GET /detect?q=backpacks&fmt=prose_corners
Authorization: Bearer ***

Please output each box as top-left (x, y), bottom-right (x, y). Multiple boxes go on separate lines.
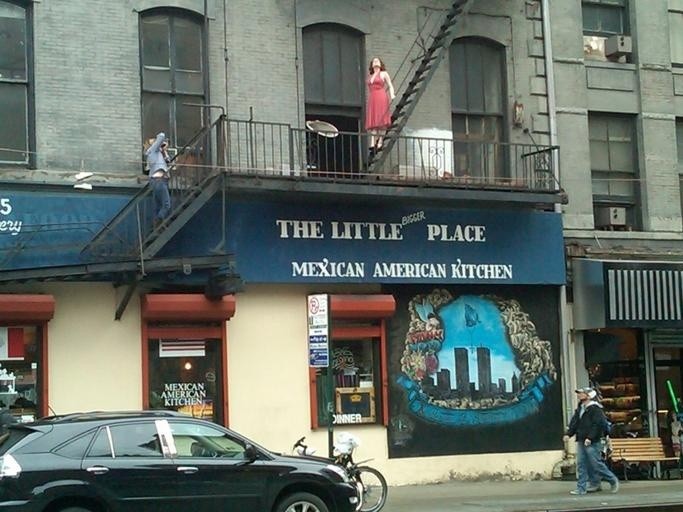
top-left (601, 416), bottom-right (613, 436)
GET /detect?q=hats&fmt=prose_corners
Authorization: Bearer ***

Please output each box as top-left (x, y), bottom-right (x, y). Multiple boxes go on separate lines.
top-left (575, 386), bottom-right (599, 398)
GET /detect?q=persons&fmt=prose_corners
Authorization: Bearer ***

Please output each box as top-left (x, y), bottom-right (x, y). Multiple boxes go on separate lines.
top-left (365, 55), bottom-right (396, 151)
top-left (585, 433), bottom-right (613, 493)
top-left (142, 130), bottom-right (175, 233)
top-left (567, 385), bottom-right (620, 494)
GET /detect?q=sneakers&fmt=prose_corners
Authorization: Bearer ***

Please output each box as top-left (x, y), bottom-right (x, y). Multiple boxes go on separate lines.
top-left (368, 146), bottom-right (383, 151)
top-left (151, 218), bottom-right (165, 234)
top-left (567, 483), bottom-right (601, 495)
top-left (610, 481), bottom-right (619, 494)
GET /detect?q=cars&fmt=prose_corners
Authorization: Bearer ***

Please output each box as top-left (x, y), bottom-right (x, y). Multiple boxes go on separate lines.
top-left (0, 410), bottom-right (357, 512)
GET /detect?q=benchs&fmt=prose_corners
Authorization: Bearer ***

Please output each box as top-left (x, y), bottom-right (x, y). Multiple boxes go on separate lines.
top-left (601, 436), bottom-right (681, 480)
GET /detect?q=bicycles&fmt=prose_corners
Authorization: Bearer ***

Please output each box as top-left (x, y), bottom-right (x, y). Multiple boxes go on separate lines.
top-left (284, 432), bottom-right (387, 511)
